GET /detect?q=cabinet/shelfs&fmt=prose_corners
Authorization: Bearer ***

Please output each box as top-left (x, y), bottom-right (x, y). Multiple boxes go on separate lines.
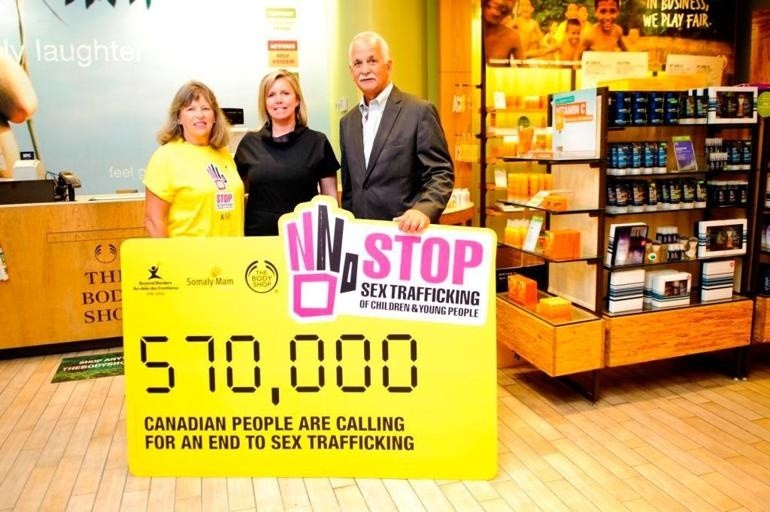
top-left (475, 77), bottom-right (770, 403)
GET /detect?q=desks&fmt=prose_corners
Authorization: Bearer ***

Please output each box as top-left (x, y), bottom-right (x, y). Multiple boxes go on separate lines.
top-left (438, 202), bottom-right (475, 226)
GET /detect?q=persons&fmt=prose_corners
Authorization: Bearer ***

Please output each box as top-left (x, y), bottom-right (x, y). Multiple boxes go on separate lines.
top-left (234, 68), bottom-right (341, 236)
top-left (338, 32), bottom-right (456, 233)
top-left (142, 79), bottom-right (247, 238)
top-left (481, 0), bottom-right (630, 64)
top-left (0, 39), bottom-right (37, 282)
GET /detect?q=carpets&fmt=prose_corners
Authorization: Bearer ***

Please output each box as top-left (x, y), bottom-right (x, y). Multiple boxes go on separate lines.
top-left (51, 352), bottom-right (124, 384)
